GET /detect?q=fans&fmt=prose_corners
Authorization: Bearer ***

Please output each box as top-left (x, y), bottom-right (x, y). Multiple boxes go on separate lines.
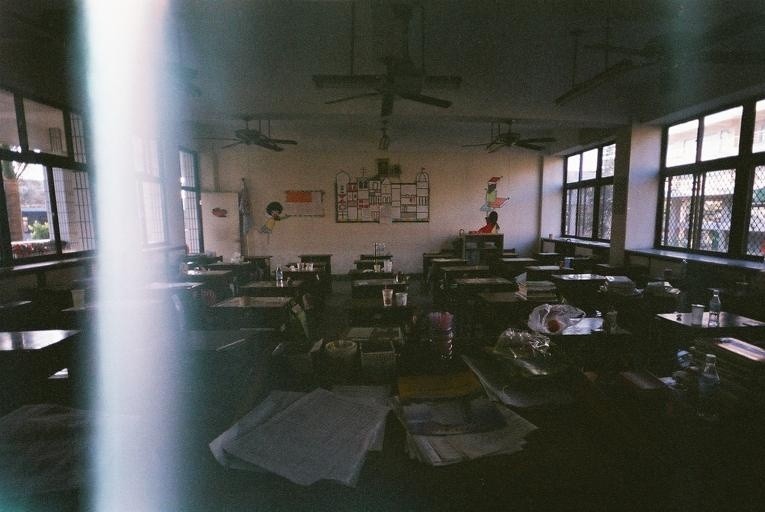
top-left (192, 116), bottom-right (299, 156)
top-left (312, 57), bottom-right (464, 119)
top-left (461, 118), bottom-right (562, 158)
top-left (595, 1), bottom-right (765, 83)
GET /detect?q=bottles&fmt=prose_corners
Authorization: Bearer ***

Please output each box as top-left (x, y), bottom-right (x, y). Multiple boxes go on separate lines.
top-left (668, 345), bottom-right (720, 423)
top-left (690, 283), bottom-right (725, 326)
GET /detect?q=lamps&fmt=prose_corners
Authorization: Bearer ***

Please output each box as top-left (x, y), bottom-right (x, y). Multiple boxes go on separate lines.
top-left (312, 0), bottom-right (465, 92)
top-left (552, 14), bottom-right (637, 108)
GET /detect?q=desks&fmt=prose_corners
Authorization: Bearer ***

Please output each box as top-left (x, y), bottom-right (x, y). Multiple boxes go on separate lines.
top-left (154, 254), bottom-right (331, 385)
top-left (423, 252), bottom-right (764, 429)
top-left (349, 255), bottom-right (412, 366)
top-left (1, 276), bottom-right (95, 404)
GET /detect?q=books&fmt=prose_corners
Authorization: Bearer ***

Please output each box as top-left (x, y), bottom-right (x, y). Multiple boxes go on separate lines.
top-left (706, 336), bottom-right (765, 412)
top-left (599, 275), bottom-right (642, 300)
top-left (397, 370), bottom-right (480, 403)
top-left (516, 279), bottom-right (558, 304)
top-left (618, 368), bottom-right (667, 400)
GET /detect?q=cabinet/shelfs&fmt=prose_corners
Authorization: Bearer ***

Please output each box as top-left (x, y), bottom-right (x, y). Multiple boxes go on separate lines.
top-left (461, 232), bottom-right (505, 264)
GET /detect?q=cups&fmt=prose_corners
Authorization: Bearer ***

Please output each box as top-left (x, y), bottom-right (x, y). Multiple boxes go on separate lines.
top-left (187, 253), bottom-right (314, 286)
top-left (373, 257), bottom-right (410, 309)
top-left (68, 289), bottom-right (86, 310)
